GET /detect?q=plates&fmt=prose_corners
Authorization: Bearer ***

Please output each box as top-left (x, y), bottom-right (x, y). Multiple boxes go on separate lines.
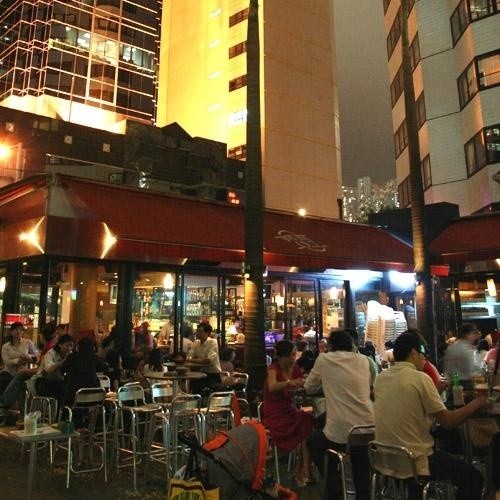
top-left (176, 367), bottom-right (188, 372)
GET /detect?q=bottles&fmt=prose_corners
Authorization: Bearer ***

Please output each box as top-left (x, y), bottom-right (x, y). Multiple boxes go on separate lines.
top-left (453, 371), bottom-right (464, 408)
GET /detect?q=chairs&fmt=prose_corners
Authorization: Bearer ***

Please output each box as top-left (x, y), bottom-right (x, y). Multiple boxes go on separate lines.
top-left (52, 388), bottom-right (107, 489)
top-left (152, 382), bottom-right (183, 412)
top-left (200, 391), bottom-right (234, 446)
top-left (108, 384), bottom-right (164, 489)
top-left (368, 440), bottom-right (458, 500)
top-left (322, 425), bottom-right (375, 499)
top-left (213, 371), bottom-right (252, 433)
top-left (119, 382), bottom-right (141, 449)
top-left (220, 371), bottom-right (230, 383)
top-left (98, 375), bottom-right (118, 442)
top-left (258, 400), bottom-right (296, 484)
top-left (141, 394), bottom-right (202, 491)
top-left (22, 389), bottom-right (57, 464)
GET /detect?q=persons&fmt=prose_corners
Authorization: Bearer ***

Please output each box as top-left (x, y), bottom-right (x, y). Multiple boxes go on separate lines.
top-left (264, 322), bottom-right (499, 499)
top-left (0, 313), bottom-right (244, 452)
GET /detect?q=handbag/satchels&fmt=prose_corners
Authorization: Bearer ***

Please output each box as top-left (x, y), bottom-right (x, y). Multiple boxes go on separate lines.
top-left (167, 436), bottom-right (220, 500)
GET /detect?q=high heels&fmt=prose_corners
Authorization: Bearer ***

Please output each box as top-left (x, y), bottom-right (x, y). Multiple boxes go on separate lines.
top-left (292, 466), bottom-right (316, 489)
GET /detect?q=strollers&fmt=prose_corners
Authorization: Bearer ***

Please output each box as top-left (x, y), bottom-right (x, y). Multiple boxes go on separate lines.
top-left (167, 420), bottom-right (301, 495)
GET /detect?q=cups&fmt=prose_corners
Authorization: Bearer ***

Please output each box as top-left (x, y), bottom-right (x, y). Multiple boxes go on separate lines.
top-left (24, 415), bottom-right (36, 435)
top-left (473, 384), bottom-right (489, 412)
top-left (163, 362), bottom-right (176, 375)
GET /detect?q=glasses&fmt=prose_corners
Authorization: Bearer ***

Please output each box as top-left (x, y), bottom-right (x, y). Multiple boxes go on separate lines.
top-left (418, 350), bottom-right (429, 359)
